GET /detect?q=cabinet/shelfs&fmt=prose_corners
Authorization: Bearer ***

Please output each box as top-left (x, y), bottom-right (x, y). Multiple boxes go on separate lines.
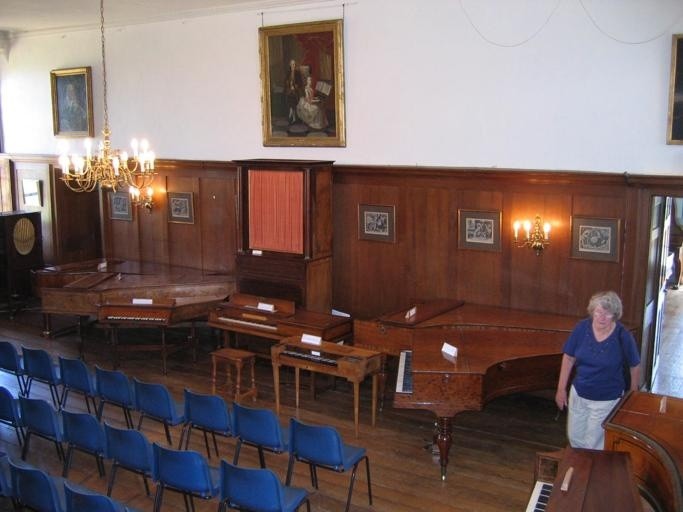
top-left (234, 252), bottom-right (307, 300)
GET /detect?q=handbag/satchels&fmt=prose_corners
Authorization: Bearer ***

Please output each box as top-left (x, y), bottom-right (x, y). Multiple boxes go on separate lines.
top-left (624, 360), bottom-right (631, 391)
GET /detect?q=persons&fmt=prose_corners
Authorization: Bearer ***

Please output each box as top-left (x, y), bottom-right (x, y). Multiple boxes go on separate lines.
top-left (285, 59), bottom-right (306, 126)
top-left (296, 77), bottom-right (328, 129)
top-left (555, 291), bottom-right (640, 451)
top-left (59, 84), bottom-right (87, 131)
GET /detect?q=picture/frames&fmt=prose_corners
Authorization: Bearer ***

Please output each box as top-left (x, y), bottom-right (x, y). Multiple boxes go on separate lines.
top-left (107, 192), bottom-right (133, 221)
top-left (166, 190), bottom-right (195, 225)
top-left (50, 66), bottom-right (95, 138)
top-left (457, 209), bottom-right (502, 254)
top-left (667, 35), bottom-right (683, 146)
top-left (358, 204), bottom-right (396, 245)
top-left (569, 216), bottom-right (622, 263)
top-left (20, 178), bottom-right (43, 208)
top-left (259, 19), bottom-right (347, 148)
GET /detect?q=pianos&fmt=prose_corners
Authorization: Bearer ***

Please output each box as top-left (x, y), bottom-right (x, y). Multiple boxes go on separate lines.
top-left (270, 334), bottom-right (384, 439)
top-left (98, 296), bottom-right (228, 375)
top-left (355, 297), bottom-right (639, 482)
top-left (523, 447), bottom-right (638, 511)
top-left (32, 256), bottom-right (235, 344)
top-left (208, 293), bottom-right (352, 400)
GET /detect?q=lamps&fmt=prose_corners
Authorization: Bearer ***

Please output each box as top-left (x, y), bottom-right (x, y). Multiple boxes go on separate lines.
top-left (57, 0), bottom-right (157, 193)
top-left (512, 210), bottom-right (552, 256)
top-left (130, 186), bottom-right (156, 212)
